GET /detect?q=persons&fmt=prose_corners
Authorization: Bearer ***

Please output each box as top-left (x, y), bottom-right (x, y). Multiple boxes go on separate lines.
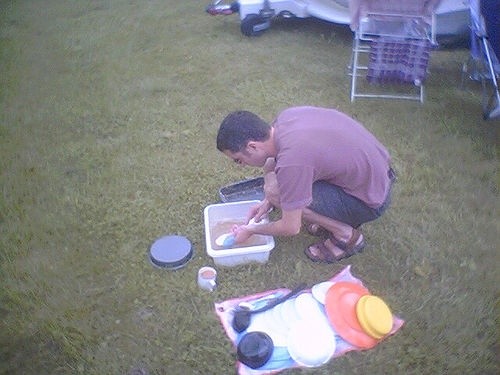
top-left (212, 105), bottom-right (396, 263)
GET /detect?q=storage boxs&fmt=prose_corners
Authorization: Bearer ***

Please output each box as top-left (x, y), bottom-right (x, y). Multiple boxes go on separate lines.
top-left (204, 199), bottom-right (275, 268)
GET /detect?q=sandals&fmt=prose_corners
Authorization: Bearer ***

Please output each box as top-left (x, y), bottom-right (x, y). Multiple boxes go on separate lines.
top-left (307, 224), bottom-right (361, 238)
top-left (304, 228), bottom-right (367, 263)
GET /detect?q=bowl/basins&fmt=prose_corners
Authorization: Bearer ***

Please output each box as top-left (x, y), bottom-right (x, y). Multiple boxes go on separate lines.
top-left (236, 331), bottom-right (275, 370)
top-left (285, 319), bottom-right (336, 366)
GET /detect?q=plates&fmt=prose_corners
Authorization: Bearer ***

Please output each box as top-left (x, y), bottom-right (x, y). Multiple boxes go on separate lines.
top-left (149, 236), bottom-right (193, 269)
top-left (232, 281), bottom-right (394, 370)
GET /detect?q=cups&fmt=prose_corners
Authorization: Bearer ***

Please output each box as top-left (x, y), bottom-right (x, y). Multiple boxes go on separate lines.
top-left (198, 267), bottom-right (217, 292)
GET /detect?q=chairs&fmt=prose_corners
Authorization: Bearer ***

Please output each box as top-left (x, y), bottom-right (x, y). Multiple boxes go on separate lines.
top-left (346, 0), bottom-right (441, 104)
